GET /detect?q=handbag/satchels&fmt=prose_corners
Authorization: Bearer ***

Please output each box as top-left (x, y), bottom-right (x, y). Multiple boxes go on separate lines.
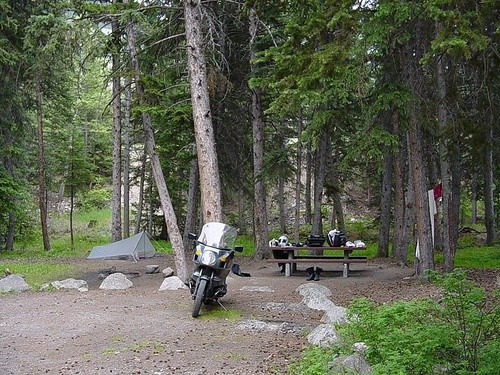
top-left (327, 228), bottom-right (346, 247)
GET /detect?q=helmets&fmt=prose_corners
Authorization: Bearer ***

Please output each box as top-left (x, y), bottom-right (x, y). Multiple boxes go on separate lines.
top-left (278, 236), bottom-right (288, 246)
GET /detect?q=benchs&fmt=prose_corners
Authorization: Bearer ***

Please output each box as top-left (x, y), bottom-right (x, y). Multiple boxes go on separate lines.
top-left (293, 256), bottom-right (367, 273)
top-left (266, 259), bottom-right (367, 278)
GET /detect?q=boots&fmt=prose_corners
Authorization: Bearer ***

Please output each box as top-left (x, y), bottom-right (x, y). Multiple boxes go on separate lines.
top-left (314, 267), bottom-right (322, 281)
top-left (306, 267), bottom-right (315, 281)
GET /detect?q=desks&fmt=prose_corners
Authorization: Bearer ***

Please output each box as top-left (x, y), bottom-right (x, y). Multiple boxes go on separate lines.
top-left (266, 246), bottom-right (368, 274)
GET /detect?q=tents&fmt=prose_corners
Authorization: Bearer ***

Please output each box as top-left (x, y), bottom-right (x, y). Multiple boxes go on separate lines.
top-left (84, 230), bottom-right (156, 261)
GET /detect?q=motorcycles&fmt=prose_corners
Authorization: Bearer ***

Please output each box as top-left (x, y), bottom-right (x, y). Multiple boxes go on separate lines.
top-left (184, 222), bottom-right (243, 318)
